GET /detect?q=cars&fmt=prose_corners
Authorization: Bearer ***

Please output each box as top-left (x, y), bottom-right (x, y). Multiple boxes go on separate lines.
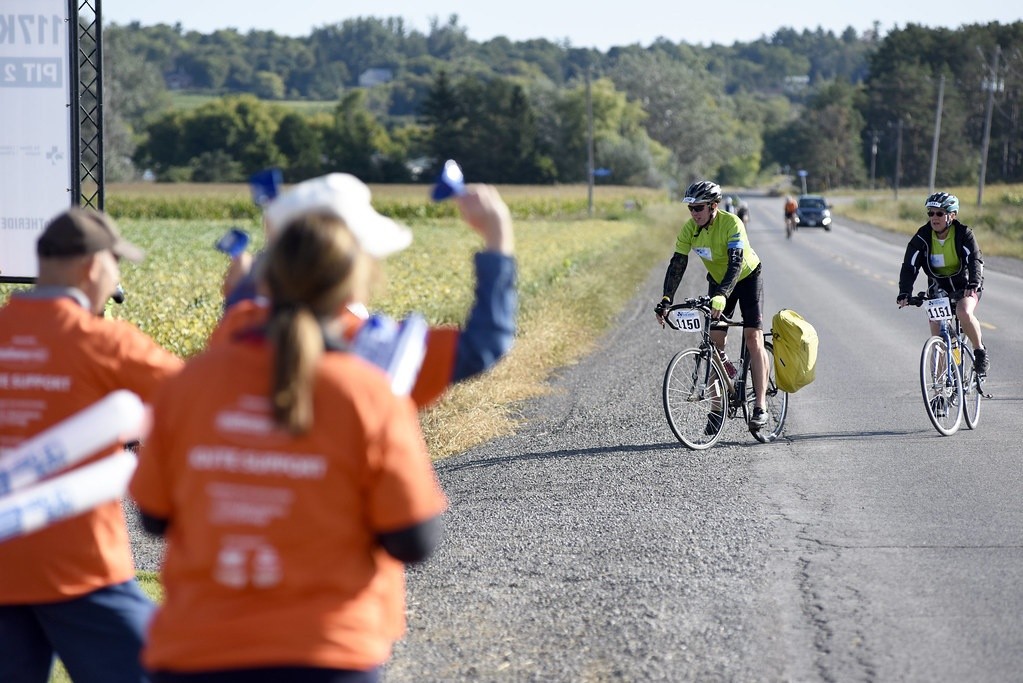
top-left (795, 195), bottom-right (834, 232)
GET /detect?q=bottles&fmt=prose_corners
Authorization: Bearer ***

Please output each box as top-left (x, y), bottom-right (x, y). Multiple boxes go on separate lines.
top-left (716, 347), bottom-right (737, 378)
top-left (951, 335), bottom-right (961, 365)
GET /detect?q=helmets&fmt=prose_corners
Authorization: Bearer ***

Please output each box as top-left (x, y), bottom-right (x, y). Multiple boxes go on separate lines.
top-left (681, 181), bottom-right (722, 203)
top-left (925, 192), bottom-right (959, 215)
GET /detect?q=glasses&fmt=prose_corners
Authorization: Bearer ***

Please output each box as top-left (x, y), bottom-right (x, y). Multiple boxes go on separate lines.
top-left (688, 203), bottom-right (713, 212)
top-left (927, 211), bottom-right (951, 217)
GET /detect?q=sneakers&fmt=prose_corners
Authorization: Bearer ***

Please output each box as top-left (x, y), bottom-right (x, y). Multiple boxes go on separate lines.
top-left (930, 396), bottom-right (945, 417)
top-left (748, 405), bottom-right (769, 427)
top-left (704, 411), bottom-right (724, 435)
top-left (974, 344), bottom-right (990, 374)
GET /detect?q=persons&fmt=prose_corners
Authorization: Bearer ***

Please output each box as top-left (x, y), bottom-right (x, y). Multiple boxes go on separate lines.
top-left (896, 192), bottom-right (990, 417)
top-left (784, 197), bottom-right (798, 234)
top-left (724, 196), bottom-right (750, 224)
top-left (656, 180), bottom-right (769, 435)
top-left (0, 205), bottom-right (185, 683)
top-left (129, 172), bottom-right (517, 683)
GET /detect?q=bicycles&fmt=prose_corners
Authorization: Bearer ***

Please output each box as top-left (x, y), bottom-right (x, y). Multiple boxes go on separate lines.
top-left (653, 294), bottom-right (790, 451)
top-left (783, 211), bottom-right (798, 238)
top-left (896, 286), bottom-right (993, 437)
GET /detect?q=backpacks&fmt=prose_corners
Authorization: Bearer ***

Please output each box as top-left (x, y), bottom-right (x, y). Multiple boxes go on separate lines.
top-left (771, 310), bottom-right (818, 393)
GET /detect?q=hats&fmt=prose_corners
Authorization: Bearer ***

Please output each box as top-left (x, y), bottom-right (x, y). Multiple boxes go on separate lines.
top-left (264, 173), bottom-right (414, 257)
top-left (38, 208), bottom-right (144, 262)
